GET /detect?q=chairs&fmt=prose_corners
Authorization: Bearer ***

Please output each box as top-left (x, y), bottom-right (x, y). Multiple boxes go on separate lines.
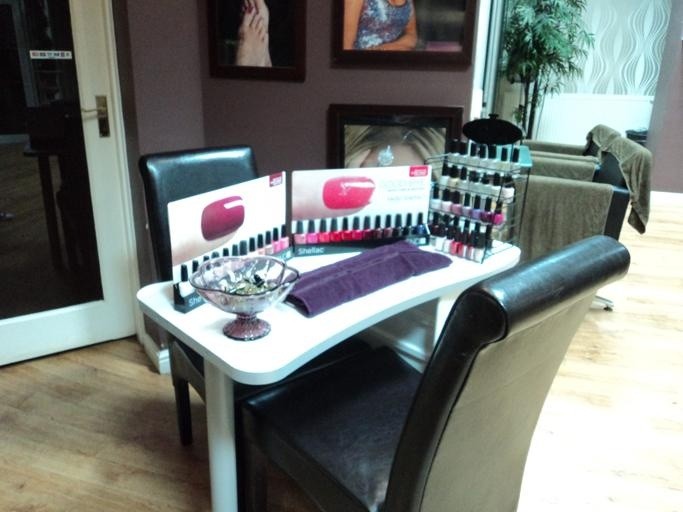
top-left (139, 147), bottom-right (373, 487)
top-left (510, 125), bottom-right (651, 266)
top-left (261, 234), bottom-right (631, 512)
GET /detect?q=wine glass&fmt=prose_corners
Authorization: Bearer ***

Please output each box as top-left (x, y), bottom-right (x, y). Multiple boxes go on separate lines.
top-left (189, 255), bottom-right (299, 341)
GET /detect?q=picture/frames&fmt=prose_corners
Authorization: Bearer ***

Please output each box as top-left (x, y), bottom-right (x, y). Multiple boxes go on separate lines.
top-left (207, 0), bottom-right (477, 83)
top-left (327, 104), bottom-right (463, 167)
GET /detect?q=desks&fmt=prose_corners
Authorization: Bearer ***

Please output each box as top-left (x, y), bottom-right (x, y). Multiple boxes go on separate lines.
top-left (137, 223), bottom-right (522, 512)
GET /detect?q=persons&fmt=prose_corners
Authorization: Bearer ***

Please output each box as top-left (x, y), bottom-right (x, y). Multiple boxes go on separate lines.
top-left (232, 0), bottom-right (272, 67)
top-left (345, 123), bottom-right (446, 168)
top-left (342, 0), bottom-right (417, 50)
